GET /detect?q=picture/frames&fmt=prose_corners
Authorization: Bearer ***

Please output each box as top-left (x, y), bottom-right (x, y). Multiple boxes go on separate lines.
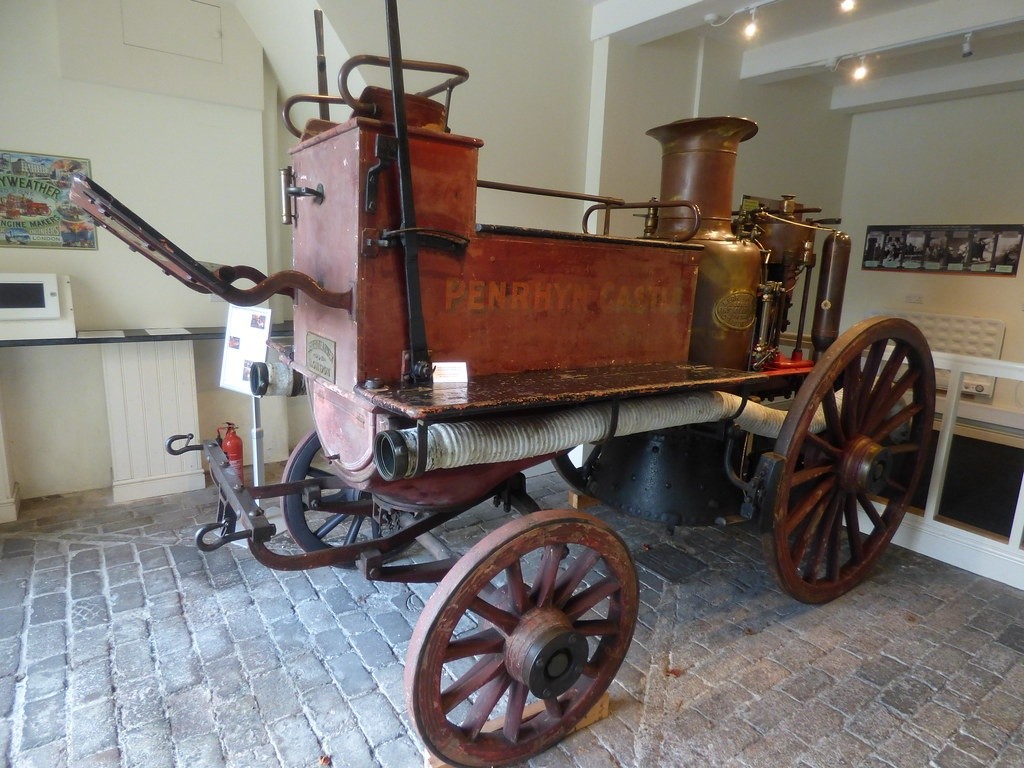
top-left (860, 224), bottom-right (1024, 274)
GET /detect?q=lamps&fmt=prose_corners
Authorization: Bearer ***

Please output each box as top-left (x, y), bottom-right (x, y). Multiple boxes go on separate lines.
top-left (853, 55), bottom-right (868, 81)
top-left (744, 8), bottom-right (759, 38)
top-left (961, 32), bottom-right (973, 58)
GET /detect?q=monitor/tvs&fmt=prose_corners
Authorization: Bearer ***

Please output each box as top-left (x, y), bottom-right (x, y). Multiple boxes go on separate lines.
top-left (0, 274), bottom-right (60, 321)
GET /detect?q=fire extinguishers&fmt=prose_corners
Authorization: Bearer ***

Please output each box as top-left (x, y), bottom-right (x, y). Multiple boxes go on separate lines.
top-left (216, 421), bottom-right (244, 487)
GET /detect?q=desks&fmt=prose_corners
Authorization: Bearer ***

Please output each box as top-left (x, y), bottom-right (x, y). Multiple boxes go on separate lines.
top-left (0, 320), bottom-right (294, 523)
